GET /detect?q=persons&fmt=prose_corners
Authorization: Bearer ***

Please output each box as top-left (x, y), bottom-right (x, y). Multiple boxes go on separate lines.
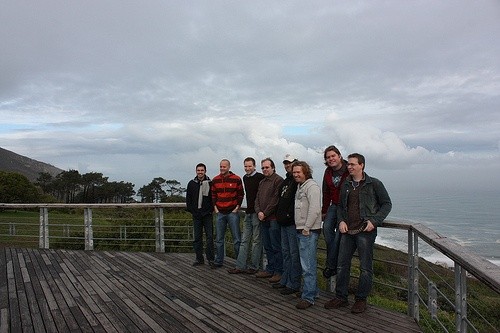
top-left (183, 144), bottom-right (392, 313)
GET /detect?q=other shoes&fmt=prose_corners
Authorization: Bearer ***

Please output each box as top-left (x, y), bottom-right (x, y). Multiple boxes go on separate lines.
top-left (324, 297), bottom-right (348, 309)
top-left (352, 300), bottom-right (367, 312)
top-left (228, 268), bottom-right (247, 274)
top-left (256, 272), bottom-right (272, 277)
top-left (245, 269), bottom-right (258, 274)
top-left (280, 288), bottom-right (298, 295)
top-left (296, 292), bottom-right (320, 298)
top-left (208, 260), bottom-right (224, 268)
top-left (272, 283), bottom-right (286, 288)
top-left (193, 260), bottom-right (204, 265)
top-left (295, 300), bottom-right (312, 308)
top-left (271, 274), bottom-right (282, 282)
top-left (323, 267), bottom-right (337, 279)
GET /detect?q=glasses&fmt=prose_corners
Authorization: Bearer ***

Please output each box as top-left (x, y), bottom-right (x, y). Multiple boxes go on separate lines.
top-left (261, 166), bottom-right (270, 169)
top-left (346, 163), bottom-right (358, 166)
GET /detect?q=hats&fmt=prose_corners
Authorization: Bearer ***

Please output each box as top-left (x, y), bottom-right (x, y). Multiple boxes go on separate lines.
top-left (283, 153), bottom-right (297, 163)
